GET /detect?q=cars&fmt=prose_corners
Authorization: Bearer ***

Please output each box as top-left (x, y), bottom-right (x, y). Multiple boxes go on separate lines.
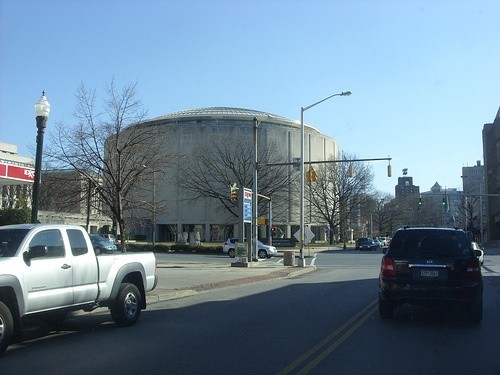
top-left (356, 236), bottom-right (392, 254)
top-left (87, 233), bottom-right (118, 255)
top-left (470, 243), bottom-right (484, 264)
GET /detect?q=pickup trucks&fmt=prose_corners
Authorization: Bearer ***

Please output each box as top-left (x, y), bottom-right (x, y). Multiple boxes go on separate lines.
top-left (0, 224), bottom-right (158, 375)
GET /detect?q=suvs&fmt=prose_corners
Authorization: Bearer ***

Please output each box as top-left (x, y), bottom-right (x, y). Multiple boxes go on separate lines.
top-left (223, 237), bottom-right (277, 258)
top-left (378, 225), bottom-right (484, 322)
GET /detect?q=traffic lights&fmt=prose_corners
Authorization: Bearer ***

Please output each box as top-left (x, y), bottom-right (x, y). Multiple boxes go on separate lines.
top-left (270, 227), bottom-right (278, 232)
top-left (418, 198), bottom-right (422, 205)
top-left (443, 198), bottom-right (447, 206)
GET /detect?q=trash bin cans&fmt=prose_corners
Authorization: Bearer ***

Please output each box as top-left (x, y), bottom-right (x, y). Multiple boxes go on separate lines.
top-left (284, 251), bottom-right (295, 266)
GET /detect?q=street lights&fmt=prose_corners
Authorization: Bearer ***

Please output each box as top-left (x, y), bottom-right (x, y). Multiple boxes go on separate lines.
top-left (297, 91), bottom-right (352, 268)
top-left (31, 90), bottom-right (51, 224)
